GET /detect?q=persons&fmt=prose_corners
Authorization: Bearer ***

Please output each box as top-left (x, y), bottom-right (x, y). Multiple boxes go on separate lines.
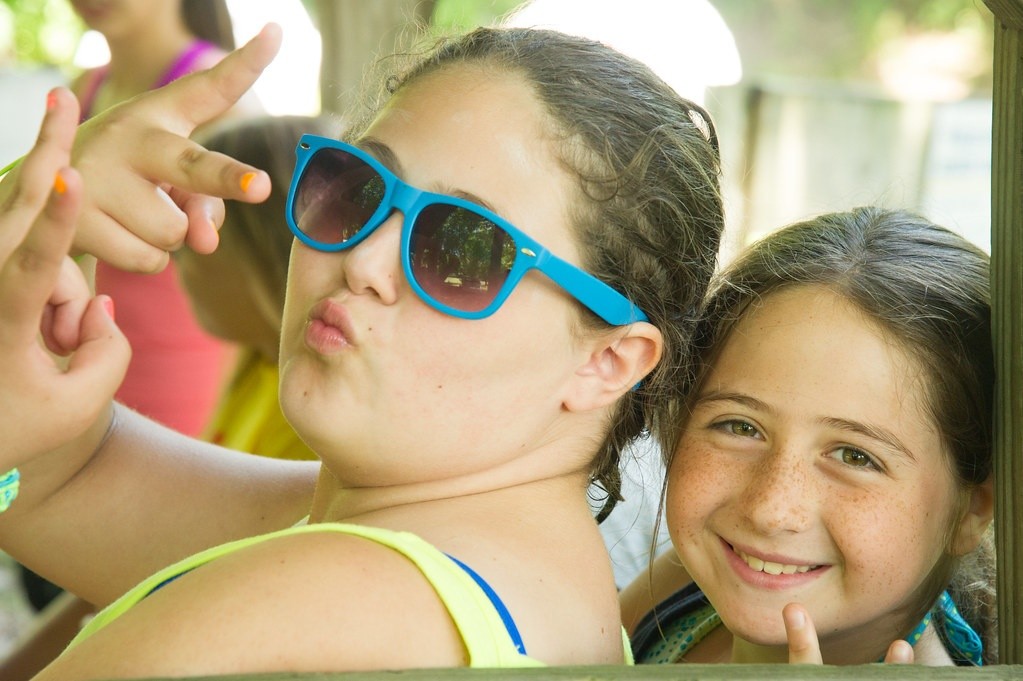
top-left (617, 205), bottom-right (997, 666)
top-left (58, 0), bottom-right (261, 437)
top-left (1, 114), bottom-right (359, 678)
top-left (2, 23), bottom-right (726, 680)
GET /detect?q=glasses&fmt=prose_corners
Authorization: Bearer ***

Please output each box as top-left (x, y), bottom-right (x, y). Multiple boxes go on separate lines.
top-left (289, 131), bottom-right (649, 397)
